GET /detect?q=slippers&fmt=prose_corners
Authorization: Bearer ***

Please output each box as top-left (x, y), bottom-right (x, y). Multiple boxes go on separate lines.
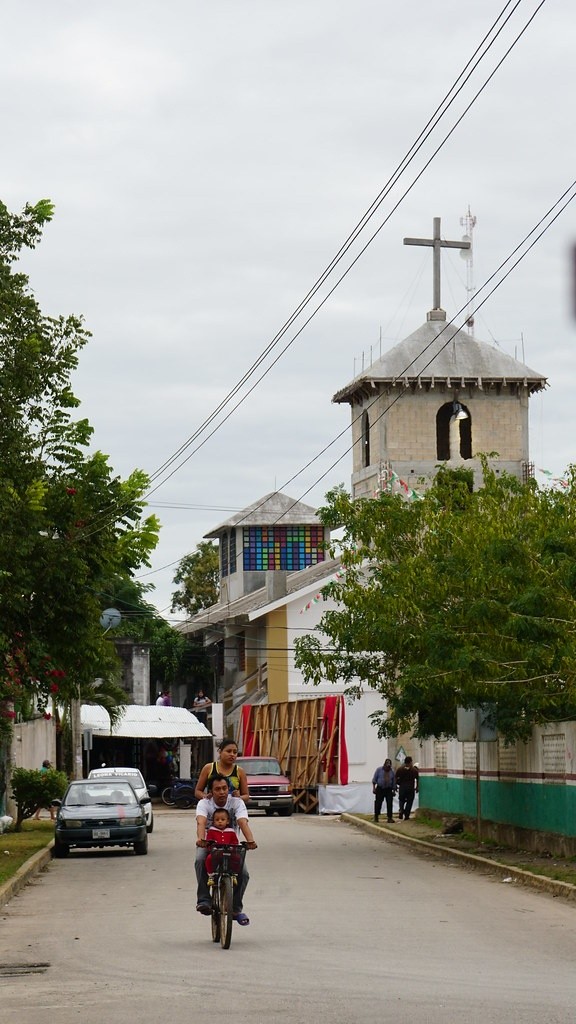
top-left (196, 901), bottom-right (213, 916)
top-left (235, 913), bottom-right (250, 926)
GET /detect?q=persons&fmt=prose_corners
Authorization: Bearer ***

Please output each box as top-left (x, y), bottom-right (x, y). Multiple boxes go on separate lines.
top-left (204, 808), bottom-right (240, 886)
top-left (156, 687), bottom-right (172, 706)
top-left (193, 690), bottom-right (211, 728)
top-left (194, 775), bottom-right (256, 926)
top-left (195, 740), bottom-right (249, 803)
top-left (372, 759), bottom-right (397, 823)
top-left (33, 760), bottom-right (57, 820)
top-left (394, 756), bottom-right (419, 820)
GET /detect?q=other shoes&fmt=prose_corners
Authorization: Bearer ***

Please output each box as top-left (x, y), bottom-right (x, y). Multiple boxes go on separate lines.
top-left (399, 812), bottom-right (403, 819)
top-left (374, 815), bottom-right (378, 822)
top-left (404, 817), bottom-right (410, 820)
top-left (388, 817), bottom-right (395, 823)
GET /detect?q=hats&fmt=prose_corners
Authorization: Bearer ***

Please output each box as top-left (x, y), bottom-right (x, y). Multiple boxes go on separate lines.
top-left (383, 759), bottom-right (391, 772)
top-left (43, 760), bottom-right (52, 765)
top-left (405, 757), bottom-right (412, 764)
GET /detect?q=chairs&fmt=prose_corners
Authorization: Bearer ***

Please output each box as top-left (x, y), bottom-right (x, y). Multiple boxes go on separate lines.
top-left (111, 791), bottom-right (124, 802)
top-left (259, 766), bottom-right (270, 772)
top-left (78, 792), bottom-right (91, 803)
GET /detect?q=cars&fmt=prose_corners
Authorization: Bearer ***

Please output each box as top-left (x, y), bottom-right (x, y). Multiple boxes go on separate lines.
top-left (87, 767), bottom-right (157, 833)
top-left (233, 756), bottom-right (295, 816)
top-left (51, 779), bottom-right (148, 857)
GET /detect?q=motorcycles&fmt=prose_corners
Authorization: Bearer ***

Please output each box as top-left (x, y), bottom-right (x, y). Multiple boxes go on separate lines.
top-left (171, 778), bottom-right (199, 810)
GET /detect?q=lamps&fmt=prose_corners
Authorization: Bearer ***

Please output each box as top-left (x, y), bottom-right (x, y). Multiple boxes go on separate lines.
top-left (453, 403), bottom-right (468, 420)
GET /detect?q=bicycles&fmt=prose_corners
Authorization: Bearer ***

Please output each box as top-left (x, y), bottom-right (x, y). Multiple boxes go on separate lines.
top-left (160, 785), bottom-right (176, 806)
top-left (198, 839), bottom-right (255, 950)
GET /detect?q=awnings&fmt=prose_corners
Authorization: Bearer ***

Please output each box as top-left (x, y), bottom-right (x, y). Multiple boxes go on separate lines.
top-left (47, 706), bottom-right (213, 738)
